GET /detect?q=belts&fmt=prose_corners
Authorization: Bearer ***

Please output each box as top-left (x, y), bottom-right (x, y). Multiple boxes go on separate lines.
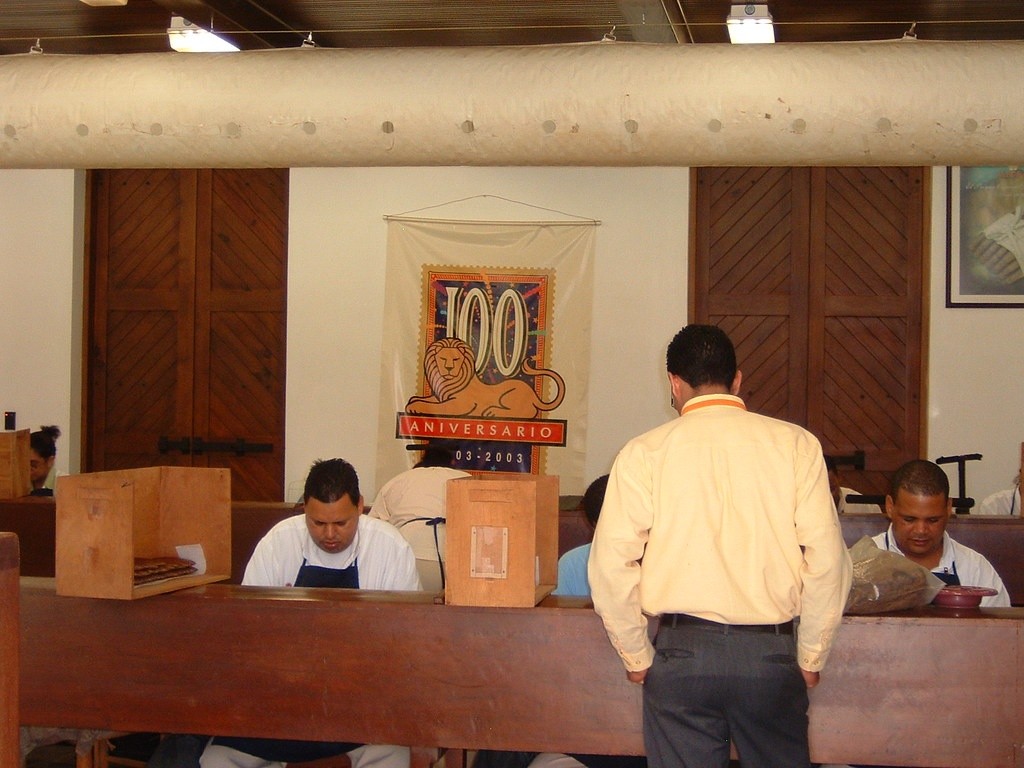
top-left (659, 613), bottom-right (794, 635)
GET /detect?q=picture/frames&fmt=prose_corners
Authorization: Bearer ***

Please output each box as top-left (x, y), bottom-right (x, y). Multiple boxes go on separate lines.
top-left (944, 165), bottom-right (1024, 309)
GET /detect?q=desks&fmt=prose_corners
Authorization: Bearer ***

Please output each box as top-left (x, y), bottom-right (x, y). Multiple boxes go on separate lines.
top-left (1, 494), bottom-right (1024, 606)
top-left (21, 578), bottom-right (1024, 768)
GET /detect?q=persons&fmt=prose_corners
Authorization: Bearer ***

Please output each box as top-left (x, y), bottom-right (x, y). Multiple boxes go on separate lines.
top-left (199, 458), bottom-right (423, 768)
top-left (366, 443), bottom-right (473, 595)
top-left (979, 471), bottom-right (1021, 515)
top-left (587, 323), bottom-right (854, 768)
top-left (29, 425), bottom-right (71, 496)
top-left (823, 454), bottom-right (883, 515)
top-left (868, 459), bottom-right (1012, 609)
top-left (529, 473), bottom-right (648, 768)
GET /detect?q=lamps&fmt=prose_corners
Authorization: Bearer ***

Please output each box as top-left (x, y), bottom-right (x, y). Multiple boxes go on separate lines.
top-left (726, 5), bottom-right (776, 43)
top-left (165, 15), bottom-right (240, 52)
